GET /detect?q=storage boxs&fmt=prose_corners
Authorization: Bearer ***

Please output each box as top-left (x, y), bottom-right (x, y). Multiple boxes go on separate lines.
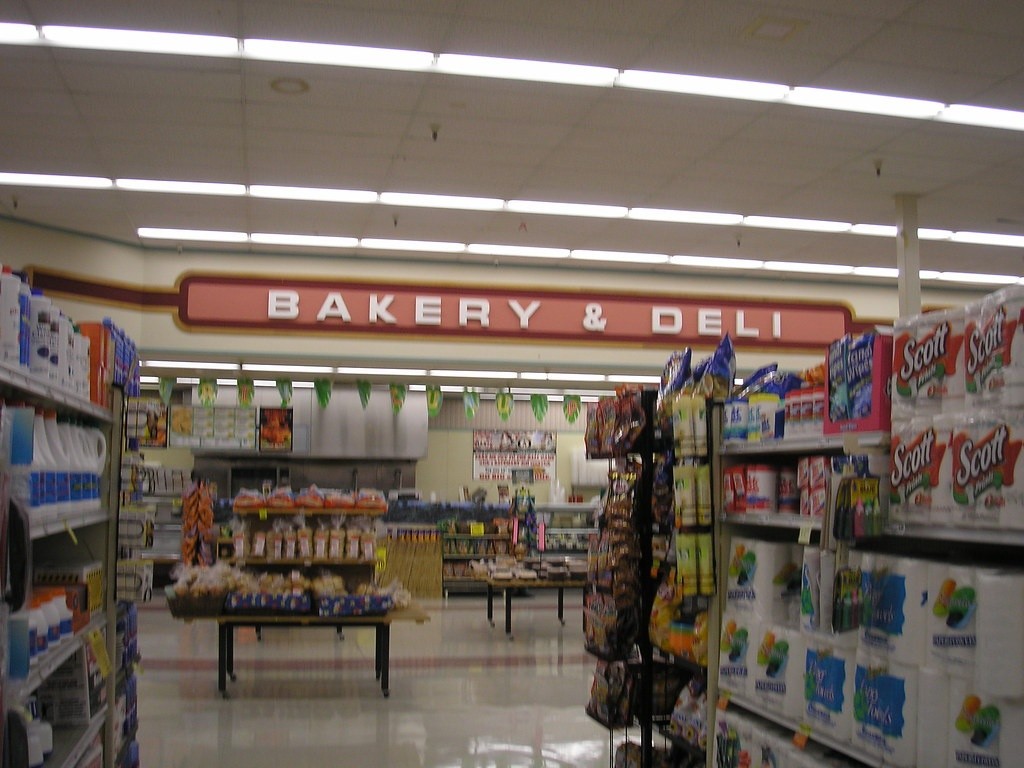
top-left (166, 585), bottom-right (394, 618)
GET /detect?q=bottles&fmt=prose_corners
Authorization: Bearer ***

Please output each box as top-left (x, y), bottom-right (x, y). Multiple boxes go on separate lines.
top-left (0, 264), bottom-right (110, 678)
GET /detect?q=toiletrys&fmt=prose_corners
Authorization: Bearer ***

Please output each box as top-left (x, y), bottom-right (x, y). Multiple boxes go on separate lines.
top-left (0, 264), bottom-right (140, 768)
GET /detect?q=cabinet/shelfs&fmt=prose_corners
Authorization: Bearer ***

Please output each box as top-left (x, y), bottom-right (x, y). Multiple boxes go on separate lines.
top-left (0, 365), bottom-right (127, 768)
top-left (585, 389), bottom-right (1024, 768)
top-left (442, 504), bottom-right (597, 599)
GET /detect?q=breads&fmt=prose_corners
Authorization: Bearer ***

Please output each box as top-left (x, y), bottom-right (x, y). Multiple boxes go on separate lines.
top-left (171, 528), bottom-right (378, 598)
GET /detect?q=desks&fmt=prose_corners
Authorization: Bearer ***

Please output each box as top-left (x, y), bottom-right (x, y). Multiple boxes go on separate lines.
top-left (483, 577), bottom-right (586, 633)
top-left (184, 602), bottom-right (432, 700)
top-left (232, 507), bottom-right (384, 585)
